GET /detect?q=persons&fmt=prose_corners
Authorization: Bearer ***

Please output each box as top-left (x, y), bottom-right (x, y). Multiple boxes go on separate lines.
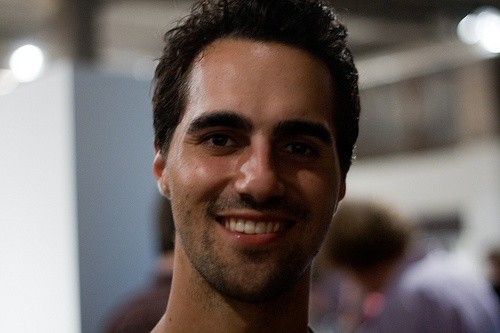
top-left (147, 1), bottom-right (361, 333)
top-left (308, 198), bottom-right (500, 332)
top-left (93, 191), bottom-right (175, 333)
